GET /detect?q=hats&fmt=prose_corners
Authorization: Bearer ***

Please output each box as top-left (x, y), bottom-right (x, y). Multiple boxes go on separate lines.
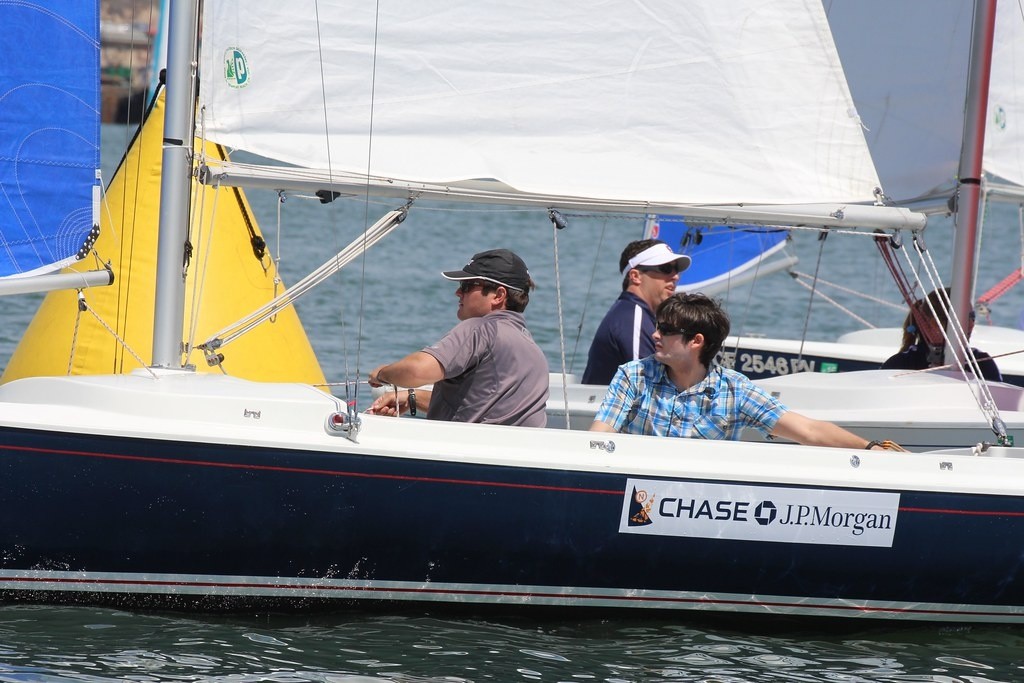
top-left (440, 248), bottom-right (530, 295)
top-left (623, 243), bottom-right (692, 281)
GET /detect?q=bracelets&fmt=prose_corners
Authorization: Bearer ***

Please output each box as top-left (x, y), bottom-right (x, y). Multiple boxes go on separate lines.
top-left (865, 439), bottom-right (881, 450)
top-left (408, 388), bottom-right (416, 416)
top-left (376, 368), bottom-right (391, 386)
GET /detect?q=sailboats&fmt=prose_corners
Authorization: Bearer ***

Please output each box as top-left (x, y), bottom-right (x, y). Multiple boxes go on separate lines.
top-left (1, 0), bottom-right (1024, 641)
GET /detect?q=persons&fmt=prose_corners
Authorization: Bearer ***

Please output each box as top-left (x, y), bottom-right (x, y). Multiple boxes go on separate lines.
top-left (581, 239), bottom-right (692, 385)
top-left (589, 293), bottom-right (910, 452)
top-left (368, 249), bottom-right (549, 427)
top-left (879, 287), bottom-right (1004, 381)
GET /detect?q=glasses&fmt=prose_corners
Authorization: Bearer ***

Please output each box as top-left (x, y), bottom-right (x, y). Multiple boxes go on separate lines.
top-left (459, 281), bottom-right (489, 293)
top-left (640, 264), bottom-right (679, 274)
top-left (656, 321), bottom-right (688, 335)
